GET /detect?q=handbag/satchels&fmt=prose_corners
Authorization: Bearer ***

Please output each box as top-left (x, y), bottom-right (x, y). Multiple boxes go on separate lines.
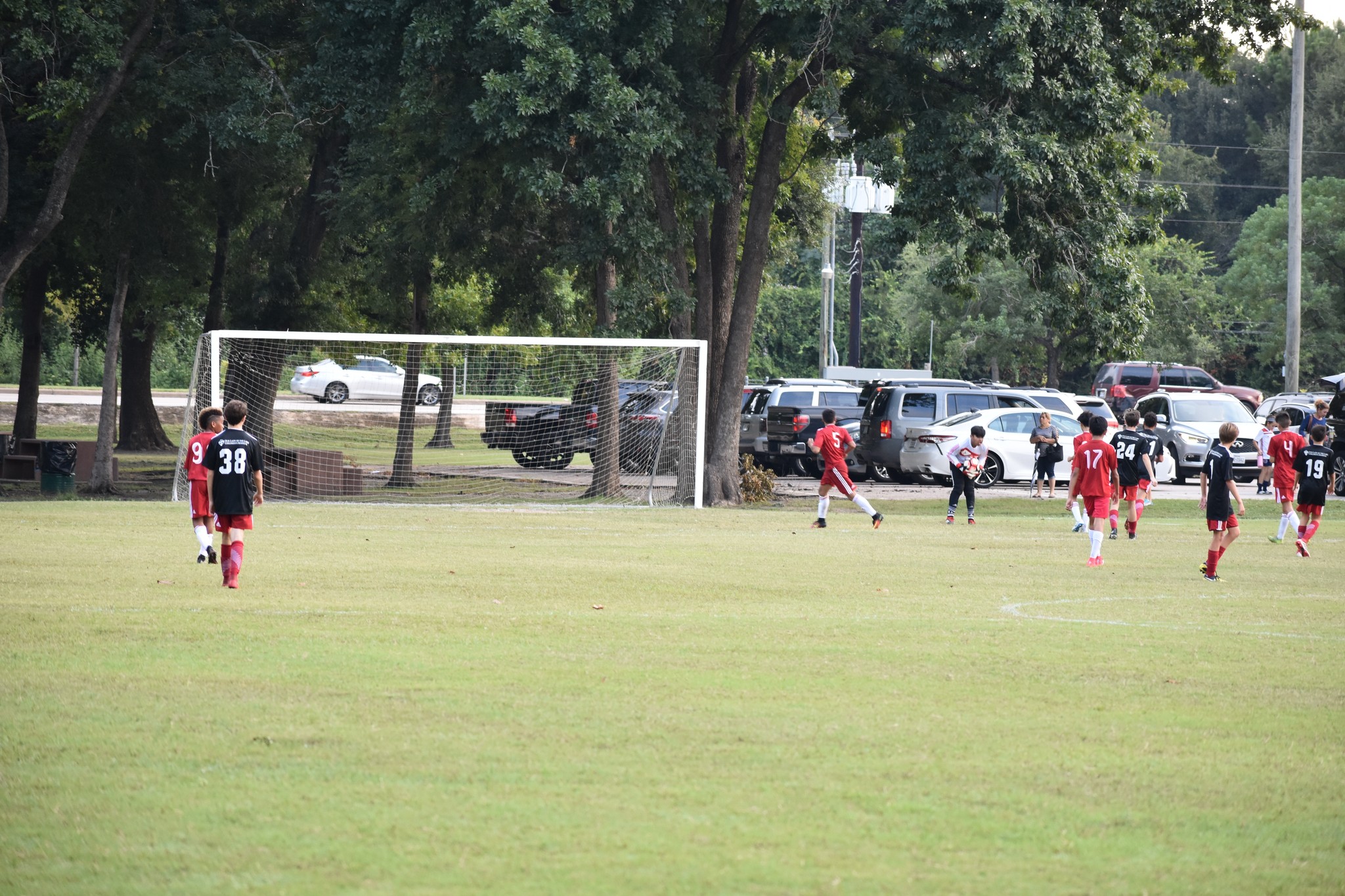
top-left (1304, 431), bottom-right (1310, 441)
top-left (1048, 446), bottom-right (1063, 462)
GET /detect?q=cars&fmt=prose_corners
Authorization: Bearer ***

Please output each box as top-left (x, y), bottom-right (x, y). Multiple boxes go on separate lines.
top-left (899, 407), bottom-right (1177, 489)
top-left (561, 374), bottom-right (1345, 496)
top-left (290, 355), bottom-right (443, 406)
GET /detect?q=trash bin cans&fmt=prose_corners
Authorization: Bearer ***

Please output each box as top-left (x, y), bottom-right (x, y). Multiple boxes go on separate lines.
top-left (40, 440), bottom-right (78, 493)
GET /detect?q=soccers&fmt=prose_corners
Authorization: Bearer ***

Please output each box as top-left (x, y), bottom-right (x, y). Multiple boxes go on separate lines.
top-left (963, 457), bottom-right (980, 474)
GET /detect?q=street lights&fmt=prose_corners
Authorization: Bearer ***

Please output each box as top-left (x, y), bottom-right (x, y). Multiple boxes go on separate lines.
top-left (841, 174), bottom-right (897, 367)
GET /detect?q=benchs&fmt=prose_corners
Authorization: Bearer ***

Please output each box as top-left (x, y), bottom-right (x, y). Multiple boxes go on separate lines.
top-left (342, 466), bottom-right (364, 496)
top-left (2, 455), bottom-right (37, 478)
top-left (263, 466), bottom-right (288, 495)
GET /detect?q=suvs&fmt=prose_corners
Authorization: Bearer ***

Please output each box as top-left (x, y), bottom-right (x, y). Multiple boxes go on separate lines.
top-left (1091, 361), bottom-right (1264, 425)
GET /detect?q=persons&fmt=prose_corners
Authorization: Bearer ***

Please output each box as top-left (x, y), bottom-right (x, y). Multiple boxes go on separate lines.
top-left (807, 409), bottom-right (883, 530)
top-left (183, 406), bottom-right (225, 564)
top-left (1252, 415), bottom-right (1277, 495)
top-left (1267, 412), bottom-right (1307, 543)
top-left (1198, 423), bottom-right (1245, 582)
top-left (945, 426), bottom-right (989, 526)
top-left (1030, 412), bottom-right (1059, 498)
top-left (1292, 424), bottom-right (1336, 558)
top-left (201, 399), bottom-right (264, 587)
top-left (1064, 408), bottom-right (1164, 567)
top-left (1299, 399), bottom-right (1330, 446)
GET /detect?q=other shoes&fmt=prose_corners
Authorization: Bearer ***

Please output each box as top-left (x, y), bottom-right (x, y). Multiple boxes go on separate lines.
top-left (1125, 516), bottom-right (1128, 530)
top-left (1032, 494), bottom-right (1041, 498)
top-left (1049, 495), bottom-right (1055, 498)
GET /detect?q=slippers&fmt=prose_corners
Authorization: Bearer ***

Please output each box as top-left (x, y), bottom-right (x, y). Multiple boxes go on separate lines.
top-left (1265, 490), bottom-right (1272, 494)
top-left (1257, 491), bottom-right (1266, 494)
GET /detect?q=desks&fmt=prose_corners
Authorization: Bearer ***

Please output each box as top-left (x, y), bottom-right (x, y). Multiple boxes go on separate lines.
top-left (261, 447), bottom-right (343, 496)
top-left (20, 439), bottom-right (96, 481)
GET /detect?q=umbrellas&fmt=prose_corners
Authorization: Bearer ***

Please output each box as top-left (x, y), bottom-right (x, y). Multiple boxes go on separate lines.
top-left (1030, 442), bottom-right (1041, 498)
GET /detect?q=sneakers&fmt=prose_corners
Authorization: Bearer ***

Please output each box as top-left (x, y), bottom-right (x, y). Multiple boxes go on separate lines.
top-left (223, 576), bottom-right (229, 586)
top-left (1143, 498), bottom-right (1153, 506)
top-left (810, 521), bottom-right (826, 528)
top-left (1071, 518), bottom-right (1084, 531)
top-left (1202, 574), bottom-right (1226, 583)
top-left (1295, 538), bottom-right (1310, 558)
top-left (1087, 559), bottom-right (1098, 567)
top-left (946, 516), bottom-right (955, 525)
top-left (1095, 555), bottom-right (1104, 565)
top-left (968, 519), bottom-right (975, 524)
top-left (1129, 532), bottom-right (1137, 541)
top-left (1109, 528), bottom-right (1117, 540)
top-left (208, 547), bottom-right (218, 564)
top-left (228, 575), bottom-right (238, 589)
top-left (1199, 562), bottom-right (1207, 574)
top-left (872, 514), bottom-right (883, 530)
top-left (1268, 535), bottom-right (1283, 544)
top-left (197, 554), bottom-right (206, 563)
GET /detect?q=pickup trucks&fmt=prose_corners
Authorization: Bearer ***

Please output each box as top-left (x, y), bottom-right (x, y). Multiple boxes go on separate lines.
top-left (480, 379), bottom-right (670, 470)
